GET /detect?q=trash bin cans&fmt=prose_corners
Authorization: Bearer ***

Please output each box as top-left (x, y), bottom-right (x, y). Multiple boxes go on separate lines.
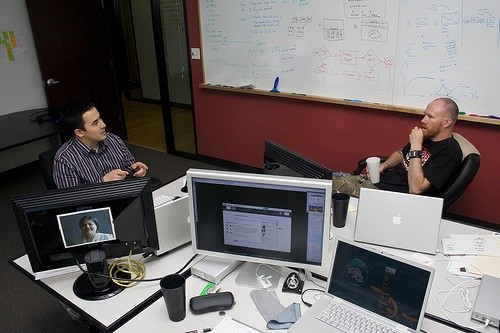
top-left (39, 149), bottom-right (57, 190)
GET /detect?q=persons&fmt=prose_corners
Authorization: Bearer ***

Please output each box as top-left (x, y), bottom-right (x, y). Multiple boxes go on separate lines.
top-left (359, 99), bottom-right (463, 198)
top-left (69, 217), bottom-right (114, 243)
top-left (52, 104), bottom-right (148, 194)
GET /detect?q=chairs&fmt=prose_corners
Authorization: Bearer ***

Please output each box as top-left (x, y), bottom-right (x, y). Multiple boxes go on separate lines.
top-left (354, 133), bottom-right (481, 208)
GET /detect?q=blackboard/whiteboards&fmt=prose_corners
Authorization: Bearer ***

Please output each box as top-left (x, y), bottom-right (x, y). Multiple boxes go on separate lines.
top-left (196, 0), bottom-right (500, 124)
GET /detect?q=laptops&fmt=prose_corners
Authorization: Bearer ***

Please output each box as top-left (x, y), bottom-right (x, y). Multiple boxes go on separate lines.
top-left (354, 187), bottom-right (444, 254)
top-left (288, 235), bottom-right (436, 333)
top-left (152, 195), bottom-right (192, 255)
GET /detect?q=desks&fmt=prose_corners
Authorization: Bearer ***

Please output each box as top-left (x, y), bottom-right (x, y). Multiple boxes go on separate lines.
top-left (11, 175), bottom-right (500, 333)
top-left (0, 107), bottom-right (63, 150)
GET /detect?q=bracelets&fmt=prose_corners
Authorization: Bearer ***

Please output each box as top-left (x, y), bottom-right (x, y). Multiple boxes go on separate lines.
top-left (408, 150), bottom-right (422, 159)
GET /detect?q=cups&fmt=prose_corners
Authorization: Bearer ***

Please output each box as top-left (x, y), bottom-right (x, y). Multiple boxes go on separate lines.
top-left (160, 274), bottom-right (187, 322)
top-left (332, 193), bottom-right (350, 228)
top-left (366, 157), bottom-right (381, 184)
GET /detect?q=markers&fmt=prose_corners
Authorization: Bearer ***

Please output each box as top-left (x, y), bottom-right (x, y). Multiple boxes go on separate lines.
top-left (291, 93), bottom-right (306, 96)
top-left (459, 111), bottom-right (500, 120)
top-left (344, 99), bottom-right (361, 102)
top-left (207, 84), bottom-right (235, 89)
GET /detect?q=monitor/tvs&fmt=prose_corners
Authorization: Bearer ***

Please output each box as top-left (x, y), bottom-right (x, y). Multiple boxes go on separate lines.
top-left (187, 168), bottom-right (333, 290)
top-left (10, 176), bottom-right (160, 300)
top-left (263, 139), bottom-right (333, 181)
